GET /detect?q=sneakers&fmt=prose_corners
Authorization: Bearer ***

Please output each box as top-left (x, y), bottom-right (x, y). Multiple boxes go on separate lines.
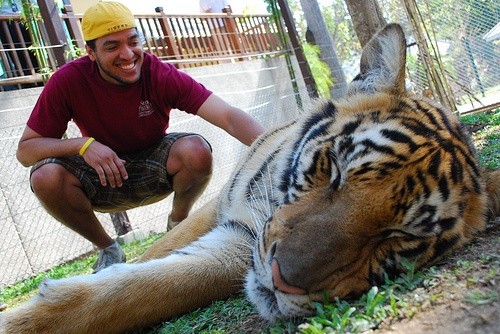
top-left (92, 241), bottom-right (126, 274)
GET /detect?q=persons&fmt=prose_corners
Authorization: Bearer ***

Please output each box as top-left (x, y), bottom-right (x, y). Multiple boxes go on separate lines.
top-left (16, 2), bottom-right (267, 274)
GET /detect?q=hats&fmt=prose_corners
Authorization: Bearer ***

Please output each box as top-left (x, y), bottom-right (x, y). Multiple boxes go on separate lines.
top-left (82, 0), bottom-right (137, 41)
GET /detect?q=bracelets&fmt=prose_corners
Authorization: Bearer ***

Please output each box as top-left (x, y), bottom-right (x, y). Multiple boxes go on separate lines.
top-left (79, 137), bottom-right (95, 156)
